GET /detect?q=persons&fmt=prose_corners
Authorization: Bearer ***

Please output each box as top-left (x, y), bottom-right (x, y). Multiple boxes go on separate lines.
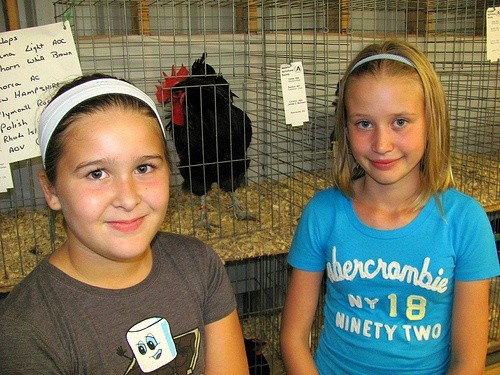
top-left (277, 38), bottom-right (500, 375)
top-left (3, 71), bottom-right (253, 375)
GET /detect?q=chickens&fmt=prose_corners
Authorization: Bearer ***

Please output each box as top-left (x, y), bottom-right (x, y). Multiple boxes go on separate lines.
top-left (155, 50), bottom-right (260, 233)
